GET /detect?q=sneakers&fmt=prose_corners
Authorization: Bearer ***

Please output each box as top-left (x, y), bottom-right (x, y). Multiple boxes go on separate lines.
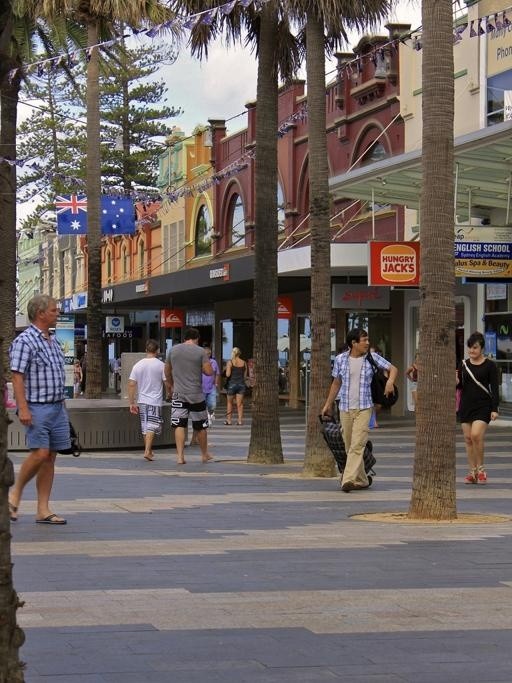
top-left (464, 473), bottom-right (476, 484)
top-left (477, 473), bottom-right (487, 484)
top-left (354, 485), bottom-right (369, 489)
top-left (341, 481), bottom-right (353, 492)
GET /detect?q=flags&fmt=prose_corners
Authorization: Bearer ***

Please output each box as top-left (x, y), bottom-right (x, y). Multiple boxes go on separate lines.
top-left (55, 194), bottom-right (137, 235)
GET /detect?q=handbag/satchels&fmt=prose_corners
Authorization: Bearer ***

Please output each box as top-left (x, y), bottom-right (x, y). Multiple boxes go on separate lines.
top-left (59, 421), bottom-right (80, 458)
top-left (366, 353), bottom-right (399, 407)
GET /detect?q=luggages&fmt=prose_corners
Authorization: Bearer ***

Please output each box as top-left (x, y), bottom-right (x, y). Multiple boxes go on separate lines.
top-left (319, 415), bottom-right (377, 477)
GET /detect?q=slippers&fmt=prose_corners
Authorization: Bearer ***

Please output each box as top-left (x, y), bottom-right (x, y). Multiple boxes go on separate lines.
top-left (36, 514), bottom-right (66, 524)
top-left (224, 421), bottom-right (232, 425)
top-left (235, 422), bottom-right (242, 425)
top-left (8, 503), bottom-right (18, 521)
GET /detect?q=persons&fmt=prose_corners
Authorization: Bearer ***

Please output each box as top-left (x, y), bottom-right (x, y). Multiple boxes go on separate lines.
top-left (74, 358), bottom-right (84, 398)
top-left (370, 345), bottom-right (381, 429)
top-left (201, 346), bottom-right (221, 428)
top-left (459, 331), bottom-right (500, 485)
top-left (319, 327), bottom-right (400, 492)
top-left (404, 353), bottom-right (417, 402)
top-left (8, 292), bottom-right (71, 525)
top-left (164, 327), bottom-right (216, 464)
top-left (127, 338), bottom-right (171, 461)
top-left (223, 346), bottom-right (248, 426)
